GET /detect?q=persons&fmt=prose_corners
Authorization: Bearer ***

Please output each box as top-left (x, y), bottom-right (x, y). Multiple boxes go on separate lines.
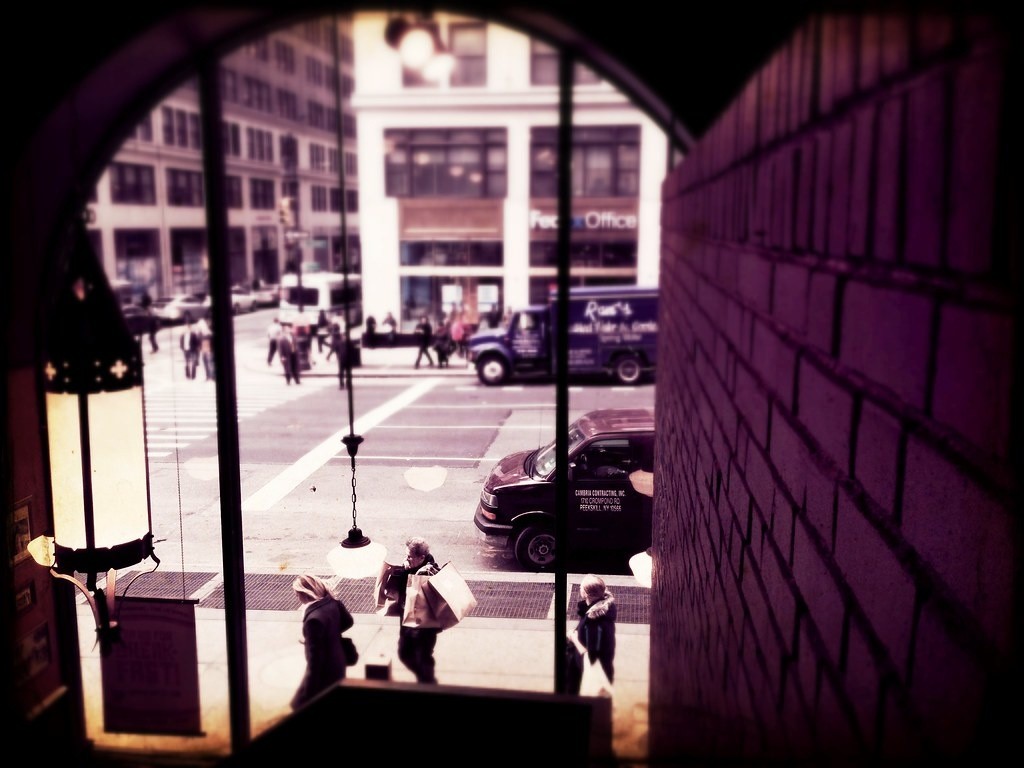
top-left (268, 302), bottom-right (512, 389)
top-left (147, 312), bottom-right (160, 354)
top-left (397, 538), bottom-right (440, 685)
top-left (290, 575), bottom-right (352, 710)
top-left (576, 576), bottom-right (616, 685)
top-left (182, 315), bottom-right (216, 381)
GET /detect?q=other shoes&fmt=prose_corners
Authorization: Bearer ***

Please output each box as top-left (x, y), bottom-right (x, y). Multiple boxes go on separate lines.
top-left (429, 360), bottom-right (434, 366)
top-left (438, 363), bottom-right (443, 368)
top-left (446, 363), bottom-right (449, 368)
top-left (415, 361), bottom-right (419, 369)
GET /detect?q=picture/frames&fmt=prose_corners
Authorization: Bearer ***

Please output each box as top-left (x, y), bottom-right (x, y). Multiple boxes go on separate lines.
top-left (21, 619), bottom-right (52, 682)
top-left (17, 577), bottom-right (38, 618)
top-left (15, 495), bottom-right (35, 568)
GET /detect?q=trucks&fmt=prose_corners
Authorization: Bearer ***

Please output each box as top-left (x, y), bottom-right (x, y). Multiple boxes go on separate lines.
top-left (465, 282), bottom-right (659, 386)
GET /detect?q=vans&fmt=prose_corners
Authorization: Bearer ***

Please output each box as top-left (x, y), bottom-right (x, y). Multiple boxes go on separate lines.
top-left (473, 406), bottom-right (656, 575)
top-left (274, 269), bottom-right (363, 338)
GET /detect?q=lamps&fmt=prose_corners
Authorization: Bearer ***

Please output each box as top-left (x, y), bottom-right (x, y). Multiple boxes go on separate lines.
top-left (23, 239), bottom-right (162, 651)
top-left (328, 13), bottom-right (385, 580)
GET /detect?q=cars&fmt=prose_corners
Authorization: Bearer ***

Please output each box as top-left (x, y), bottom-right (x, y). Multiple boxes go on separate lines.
top-left (107, 274), bottom-right (280, 334)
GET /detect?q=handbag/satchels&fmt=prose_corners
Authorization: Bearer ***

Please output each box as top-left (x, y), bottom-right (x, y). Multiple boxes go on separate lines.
top-left (422, 561), bottom-right (477, 630)
top-left (351, 346), bottom-right (361, 368)
top-left (342, 638), bottom-right (358, 666)
top-left (402, 570), bottom-right (443, 628)
top-left (374, 561), bottom-right (405, 616)
top-left (434, 339), bottom-right (456, 354)
top-left (577, 652), bottom-right (613, 699)
